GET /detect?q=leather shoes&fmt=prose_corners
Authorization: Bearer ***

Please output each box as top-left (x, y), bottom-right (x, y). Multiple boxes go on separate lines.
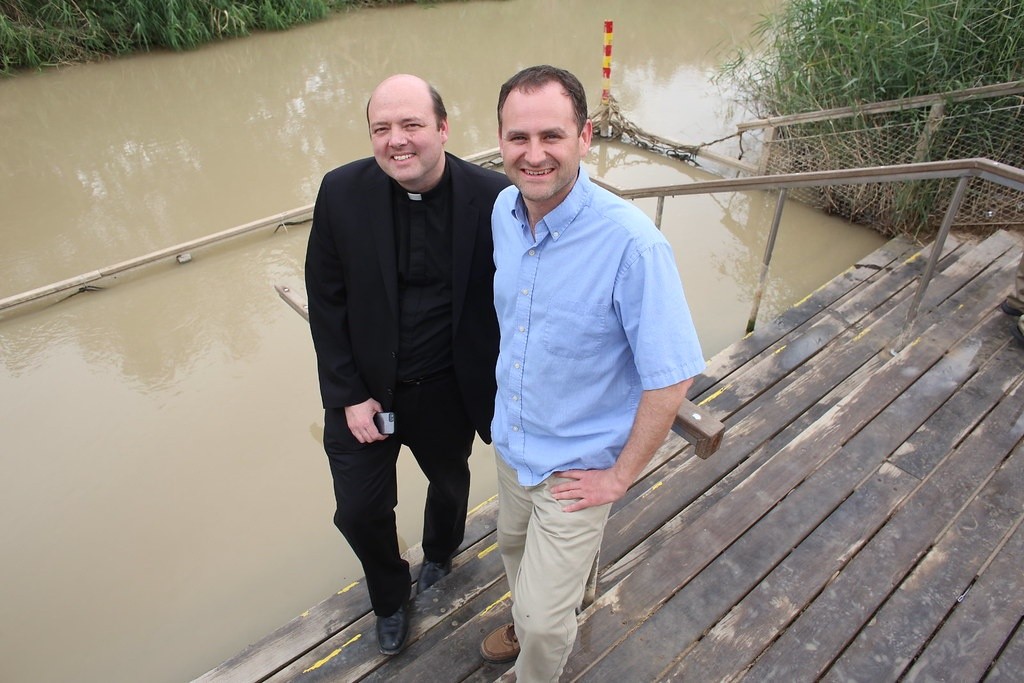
top-left (375, 571), bottom-right (412, 656)
top-left (416, 554), bottom-right (452, 594)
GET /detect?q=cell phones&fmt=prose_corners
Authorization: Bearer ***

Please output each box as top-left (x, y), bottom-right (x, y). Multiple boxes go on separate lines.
top-left (373, 411), bottom-right (395, 435)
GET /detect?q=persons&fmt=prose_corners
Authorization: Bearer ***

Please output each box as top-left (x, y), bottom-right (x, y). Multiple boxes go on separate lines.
top-left (477, 65), bottom-right (706, 683)
top-left (303, 74), bottom-right (517, 655)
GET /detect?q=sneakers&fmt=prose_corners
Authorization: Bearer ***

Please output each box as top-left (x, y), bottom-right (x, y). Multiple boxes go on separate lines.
top-left (478, 621), bottom-right (521, 664)
